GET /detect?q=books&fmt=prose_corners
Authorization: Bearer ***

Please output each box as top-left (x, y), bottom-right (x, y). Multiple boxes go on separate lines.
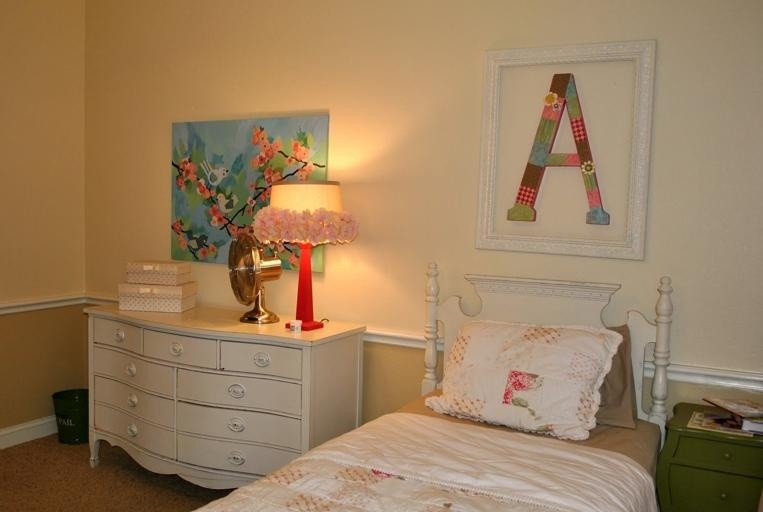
top-left (686, 397), bottom-right (763, 437)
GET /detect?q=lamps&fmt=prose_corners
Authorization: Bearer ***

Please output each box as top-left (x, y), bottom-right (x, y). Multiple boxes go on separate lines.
top-left (254, 180), bottom-right (359, 332)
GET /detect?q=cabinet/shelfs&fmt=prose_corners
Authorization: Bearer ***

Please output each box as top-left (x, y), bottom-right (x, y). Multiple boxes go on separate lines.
top-left (81, 299), bottom-right (367, 488)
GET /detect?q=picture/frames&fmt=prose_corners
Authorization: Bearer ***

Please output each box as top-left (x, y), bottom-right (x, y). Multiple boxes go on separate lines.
top-left (475, 39), bottom-right (657, 262)
top-left (170, 109), bottom-right (331, 274)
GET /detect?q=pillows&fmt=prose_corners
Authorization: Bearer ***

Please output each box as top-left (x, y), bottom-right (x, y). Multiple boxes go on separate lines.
top-left (425, 319), bottom-right (622, 441)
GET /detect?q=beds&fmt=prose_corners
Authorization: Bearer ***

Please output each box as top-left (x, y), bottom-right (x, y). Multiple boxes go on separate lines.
top-left (198, 263), bottom-right (674, 512)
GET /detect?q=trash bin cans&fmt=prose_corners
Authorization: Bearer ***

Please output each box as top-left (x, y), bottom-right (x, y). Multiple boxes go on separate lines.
top-left (51, 388), bottom-right (89, 445)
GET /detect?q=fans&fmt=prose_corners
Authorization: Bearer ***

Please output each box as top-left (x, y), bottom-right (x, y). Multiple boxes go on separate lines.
top-left (228, 232), bottom-right (282, 325)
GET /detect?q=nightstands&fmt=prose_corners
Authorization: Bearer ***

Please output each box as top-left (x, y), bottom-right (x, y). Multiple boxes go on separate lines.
top-left (653, 402), bottom-right (762, 512)
